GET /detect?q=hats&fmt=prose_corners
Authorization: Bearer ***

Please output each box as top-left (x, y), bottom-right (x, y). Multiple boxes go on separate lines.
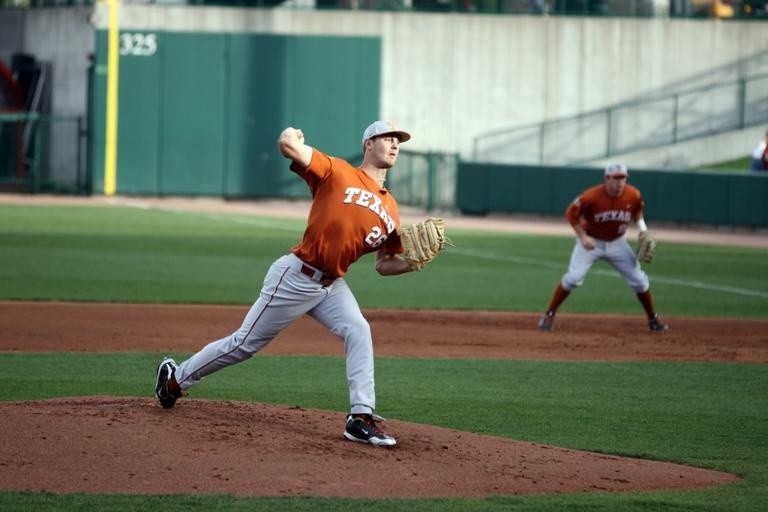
top-left (606, 162), bottom-right (628, 176)
top-left (362, 120), bottom-right (411, 143)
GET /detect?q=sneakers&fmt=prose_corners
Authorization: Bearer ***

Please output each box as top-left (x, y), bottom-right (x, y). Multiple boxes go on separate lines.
top-left (155, 356), bottom-right (189, 409)
top-left (344, 413), bottom-right (397, 447)
top-left (538, 311), bottom-right (556, 332)
top-left (648, 313), bottom-right (669, 331)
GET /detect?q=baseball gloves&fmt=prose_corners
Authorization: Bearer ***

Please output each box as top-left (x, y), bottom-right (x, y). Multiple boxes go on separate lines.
top-left (635, 231), bottom-right (657, 264)
top-left (400, 218), bottom-right (444, 271)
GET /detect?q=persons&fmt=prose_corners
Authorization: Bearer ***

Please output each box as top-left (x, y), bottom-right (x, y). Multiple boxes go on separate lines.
top-left (156, 119), bottom-right (449, 447)
top-left (537, 163), bottom-right (671, 332)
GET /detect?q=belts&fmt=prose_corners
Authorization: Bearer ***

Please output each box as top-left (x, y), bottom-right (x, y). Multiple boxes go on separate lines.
top-left (301, 265), bottom-right (335, 288)
